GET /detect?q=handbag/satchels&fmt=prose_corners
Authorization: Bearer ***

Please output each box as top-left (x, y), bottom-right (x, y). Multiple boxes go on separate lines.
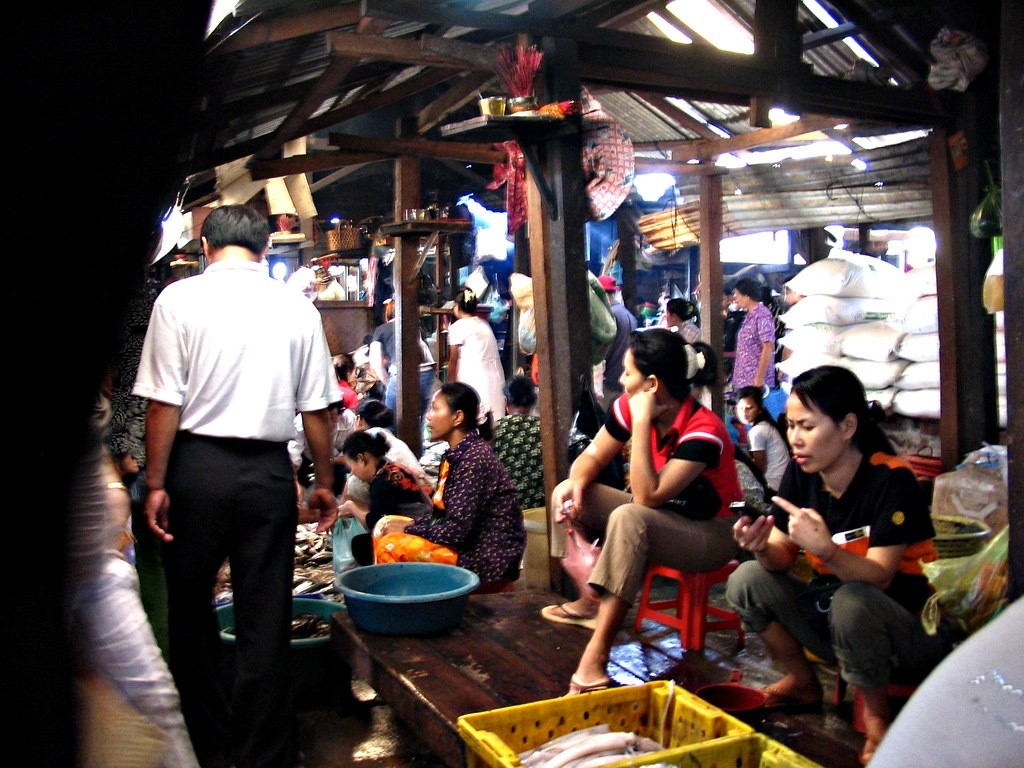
top-left (331, 513), bottom-right (371, 576)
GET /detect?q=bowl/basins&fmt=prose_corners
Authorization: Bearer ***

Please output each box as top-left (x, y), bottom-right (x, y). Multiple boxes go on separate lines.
top-left (694, 684), bottom-right (766, 719)
top-left (213, 597), bottom-right (346, 676)
top-left (924, 514), bottom-right (992, 559)
top-left (334, 561), bottom-right (480, 637)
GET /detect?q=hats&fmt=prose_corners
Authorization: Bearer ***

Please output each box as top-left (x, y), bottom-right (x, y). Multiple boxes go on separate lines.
top-left (597, 274), bottom-right (621, 290)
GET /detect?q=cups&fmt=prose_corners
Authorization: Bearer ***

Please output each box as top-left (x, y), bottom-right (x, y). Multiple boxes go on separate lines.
top-left (403, 202), bottom-right (449, 221)
top-left (477, 97), bottom-right (506, 117)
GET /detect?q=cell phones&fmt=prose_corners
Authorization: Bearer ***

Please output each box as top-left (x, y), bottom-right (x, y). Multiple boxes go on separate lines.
top-left (729, 501), bottom-right (766, 527)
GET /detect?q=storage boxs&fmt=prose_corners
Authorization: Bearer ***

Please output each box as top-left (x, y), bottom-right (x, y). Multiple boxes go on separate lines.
top-left (590, 732), bottom-right (825, 768)
top-left (457, 677), bottom-right (755, 768)
top-left (521, 506), bottom-right (552, 591)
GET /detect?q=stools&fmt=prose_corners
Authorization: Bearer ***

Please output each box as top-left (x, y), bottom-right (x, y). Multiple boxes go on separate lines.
top-left (832, 670), bottom-right (914, 734)
top-left (635, 558), bottom-right (746, 651)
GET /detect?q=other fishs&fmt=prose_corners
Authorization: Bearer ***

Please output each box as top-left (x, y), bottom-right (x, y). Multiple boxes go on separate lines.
top-left (210, 523), bottom-right (344, 637)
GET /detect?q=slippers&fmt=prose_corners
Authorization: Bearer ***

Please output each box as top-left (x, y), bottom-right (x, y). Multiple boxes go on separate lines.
top-left (540, 605), bottom-right (598, 629)
top-left (566, 676), bottom-right (612, 697)
top-left (758, 686), bottom-right (824, 707)
top-left (861, 733), bottom-right (880, 764)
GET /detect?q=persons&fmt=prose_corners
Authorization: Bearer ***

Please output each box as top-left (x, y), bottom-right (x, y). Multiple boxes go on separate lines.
top-left (334, 400), bottom-right (426, 505)
top-left (490, 374), bottom-right (544, 511)
top-left (735, 385), bottom-right (791, 504)
top-left (723, 278), bottom-right (775, 389)
top-left (597, 275), bottom-right (638, 413)
top-left (447, 289), bottom-right (507, 424)
top-left (79, 205), bottom-right (342, 768)
top-left (333, 353), bottom-right (359, 408)
top-left (725, 365), bottom-right (955, 766)
top-left (287, 399), bottom-right (358, 508)
top-left (336, 432), bottom-right (433, 566)
top-left (374, 382), bottom-right (527, 583)
top-left (541, 329), bottom-right (745, 696)
top-left (666, 298), bottom-right (701, 344)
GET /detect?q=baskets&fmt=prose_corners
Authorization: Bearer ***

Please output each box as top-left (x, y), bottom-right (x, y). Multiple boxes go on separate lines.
top-left (328, 219), bottom-right (361, 250)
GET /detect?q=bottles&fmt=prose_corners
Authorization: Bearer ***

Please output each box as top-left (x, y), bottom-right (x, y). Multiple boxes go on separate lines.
top-left (417, 268), bottom-right (439, 307)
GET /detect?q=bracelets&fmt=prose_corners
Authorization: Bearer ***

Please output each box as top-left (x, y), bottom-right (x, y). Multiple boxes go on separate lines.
top-left (106, 481), bottom-right (126, 491)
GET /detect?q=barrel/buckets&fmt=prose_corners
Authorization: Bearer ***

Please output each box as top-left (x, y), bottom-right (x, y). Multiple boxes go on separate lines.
top-left (521, 506), bottom-right (550, 589)
